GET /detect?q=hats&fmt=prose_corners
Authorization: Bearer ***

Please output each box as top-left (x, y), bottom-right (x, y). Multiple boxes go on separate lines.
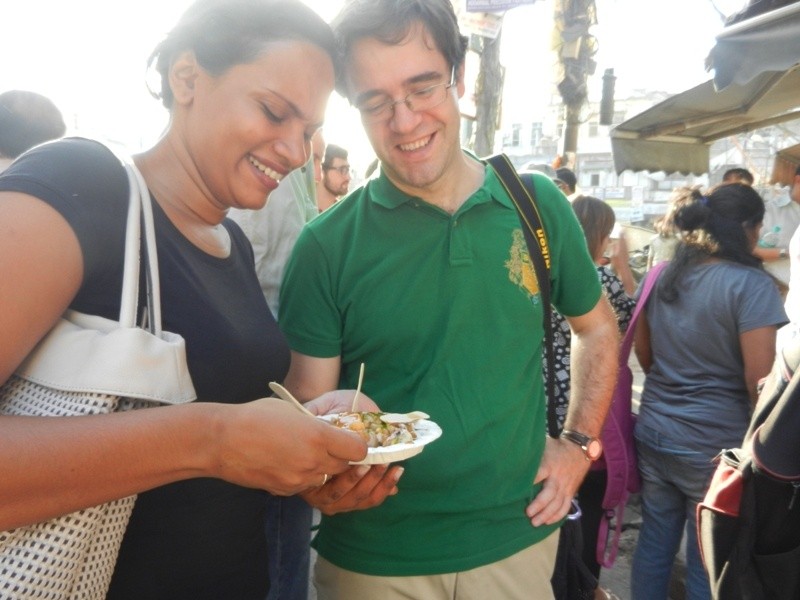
top-left (553, 168), bottom-right (576, 184)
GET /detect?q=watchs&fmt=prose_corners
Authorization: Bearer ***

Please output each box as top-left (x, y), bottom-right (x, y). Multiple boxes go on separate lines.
top-left (559, 428), bottom-right (604, 462)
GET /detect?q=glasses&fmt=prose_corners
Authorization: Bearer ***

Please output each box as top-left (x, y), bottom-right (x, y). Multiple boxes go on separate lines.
top-left (327, 165), bottom-right (353, 176)
top-left (361, 68), bottom-right (458, 124)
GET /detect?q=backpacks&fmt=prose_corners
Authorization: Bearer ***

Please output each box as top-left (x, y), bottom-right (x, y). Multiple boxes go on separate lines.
top-left (596, 258), bottom-right (642, 566)
top-left (694, 337), bottom-right (800, 600)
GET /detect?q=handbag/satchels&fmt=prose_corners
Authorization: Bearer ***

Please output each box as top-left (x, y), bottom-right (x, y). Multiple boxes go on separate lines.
top-left (550, 492), bottom-right (601, 600)
top-left (0, 134), bottom-right (198, 600)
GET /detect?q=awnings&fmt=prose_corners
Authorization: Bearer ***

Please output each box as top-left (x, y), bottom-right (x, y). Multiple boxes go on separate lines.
top-left (609, 59), bottom-right (800, 175)
top-left (706, 1), bottom-right (800, 93)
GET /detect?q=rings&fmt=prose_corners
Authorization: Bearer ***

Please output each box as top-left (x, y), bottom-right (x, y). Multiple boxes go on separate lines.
top-left (322, 473), bottom-right (328, 486)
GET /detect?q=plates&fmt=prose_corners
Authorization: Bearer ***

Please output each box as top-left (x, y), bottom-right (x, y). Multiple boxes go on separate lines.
top-left (348, 420), bottom-right (442, 465)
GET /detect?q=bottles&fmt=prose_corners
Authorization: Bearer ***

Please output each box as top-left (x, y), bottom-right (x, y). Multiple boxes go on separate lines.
top-left (758, 226), bottom-right (781, 249)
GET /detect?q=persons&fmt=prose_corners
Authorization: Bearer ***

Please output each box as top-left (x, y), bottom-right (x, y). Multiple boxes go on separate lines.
top-left (225, 124), bottom-right (381, 324)
top-left (0, 89), bottom-right (67, 176)
top-left (274, 0), bottom-right (619, 600)
top-left (0, 0), bottom-right (370, 600)
top-left (521, 164), bottom-right (800, 600)
